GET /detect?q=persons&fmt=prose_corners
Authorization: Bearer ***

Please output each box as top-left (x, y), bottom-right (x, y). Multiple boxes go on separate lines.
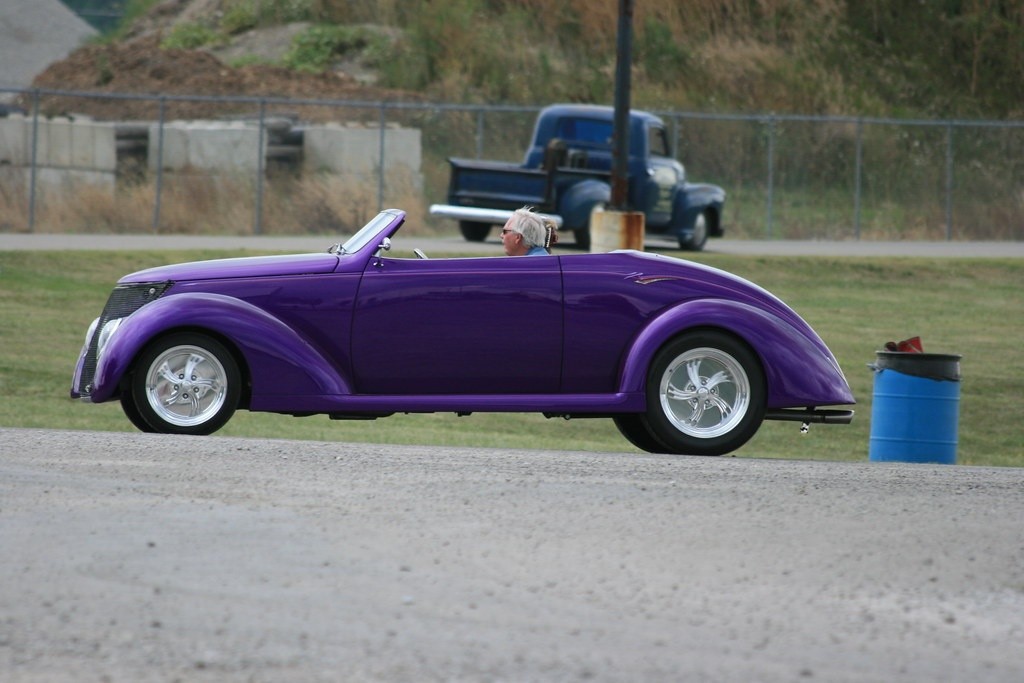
top-left (499, 205), bottom-right (560, 257)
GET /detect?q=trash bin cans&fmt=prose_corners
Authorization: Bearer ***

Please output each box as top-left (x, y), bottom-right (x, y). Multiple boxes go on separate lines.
top-left (865, 348), bottom-right (963, 467)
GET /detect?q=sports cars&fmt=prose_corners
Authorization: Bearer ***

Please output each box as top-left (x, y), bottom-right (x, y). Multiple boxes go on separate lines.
top-left (70, 203), bottom-right (857, 461)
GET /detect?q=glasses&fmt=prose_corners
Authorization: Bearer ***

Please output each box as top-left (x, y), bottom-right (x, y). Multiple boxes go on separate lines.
top-left (502, 229), bottom-right (513, 235)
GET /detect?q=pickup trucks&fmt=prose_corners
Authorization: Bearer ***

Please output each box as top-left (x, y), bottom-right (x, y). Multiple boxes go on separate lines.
top-left (430, 102), bottom-right (732, 254)
